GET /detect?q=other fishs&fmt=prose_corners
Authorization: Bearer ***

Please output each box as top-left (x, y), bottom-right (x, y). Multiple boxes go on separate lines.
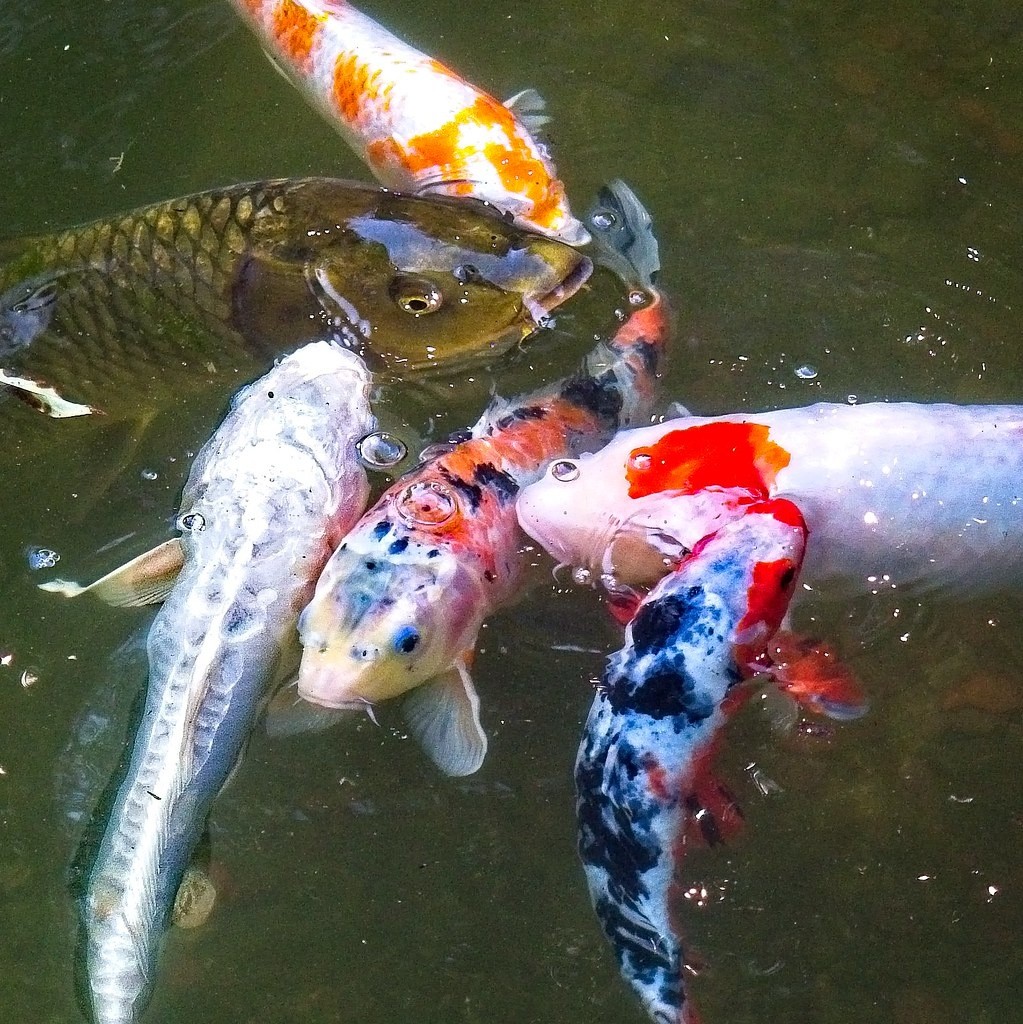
top-left (227, 0), bottom-right (596, 250)
top-left (0, 175), bottom-right (1023, 1024)
